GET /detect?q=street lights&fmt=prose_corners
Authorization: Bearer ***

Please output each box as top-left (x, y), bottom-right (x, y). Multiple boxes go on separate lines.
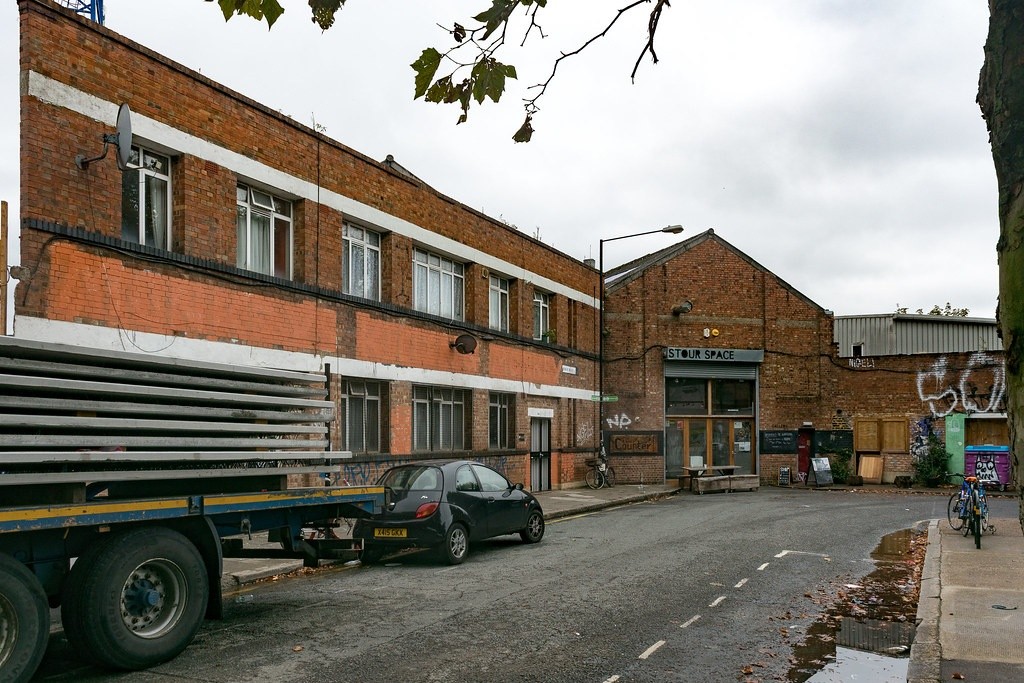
top-left (599, 224), bottom-right (684, 458)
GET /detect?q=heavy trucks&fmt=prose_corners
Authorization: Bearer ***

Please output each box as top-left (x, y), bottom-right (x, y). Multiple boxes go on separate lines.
top-left (1, 484), bottom-right (384, 681)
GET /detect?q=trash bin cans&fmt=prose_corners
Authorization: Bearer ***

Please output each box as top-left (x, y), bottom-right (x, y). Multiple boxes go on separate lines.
top-left (964, 445), bottom-right (1012, 491)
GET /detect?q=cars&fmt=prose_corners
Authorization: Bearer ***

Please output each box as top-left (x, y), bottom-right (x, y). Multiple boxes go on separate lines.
top-left (359, 459), bottom-right (544, 564)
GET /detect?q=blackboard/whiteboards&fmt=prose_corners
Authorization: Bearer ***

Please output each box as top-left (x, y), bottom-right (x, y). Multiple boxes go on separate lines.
top-left (778, 465), bottom-right (793, 486)
top-left (805, 458), bottom-right (833, 486)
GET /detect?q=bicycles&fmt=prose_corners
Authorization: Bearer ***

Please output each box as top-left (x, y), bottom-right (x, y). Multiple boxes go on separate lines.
top-left (585, 441), bottom-right (616, 490)
top-left (945, 472), bottom-right (990, 549)
